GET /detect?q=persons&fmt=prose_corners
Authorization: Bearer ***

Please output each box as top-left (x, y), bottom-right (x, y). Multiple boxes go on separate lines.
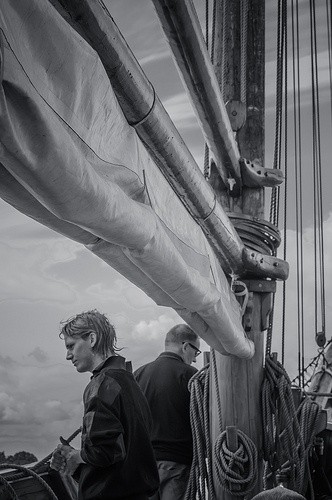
top-left (49, 309), bottom-right (161, 500)
top-left (132, 323), bottom-right (202, 500)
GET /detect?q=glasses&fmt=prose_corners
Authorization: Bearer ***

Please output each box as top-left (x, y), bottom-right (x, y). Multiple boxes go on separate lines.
top-left (180, 341), bottom-right (202, 359)
